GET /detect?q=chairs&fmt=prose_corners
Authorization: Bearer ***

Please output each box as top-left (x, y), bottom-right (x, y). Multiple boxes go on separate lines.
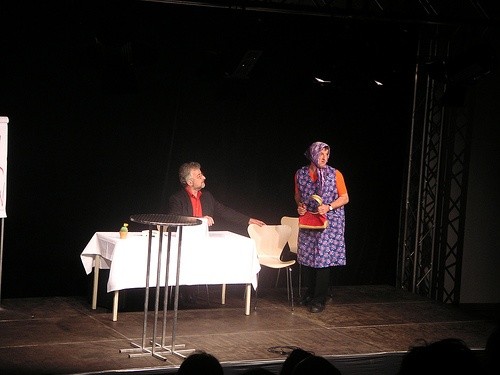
top-left (274, 216), bottom-right (302, 298)
top-left (246, 224), bottom-right (296, 311)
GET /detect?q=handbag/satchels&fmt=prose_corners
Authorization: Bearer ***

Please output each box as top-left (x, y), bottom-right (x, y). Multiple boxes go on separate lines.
top-left (299, 195), bottom-right (328, 230)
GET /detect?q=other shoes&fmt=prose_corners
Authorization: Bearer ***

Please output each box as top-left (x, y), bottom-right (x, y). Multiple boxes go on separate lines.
top-left (298, 295), bottom-right (314, 306)
top-left (310, 302), bottom-right (325, 313)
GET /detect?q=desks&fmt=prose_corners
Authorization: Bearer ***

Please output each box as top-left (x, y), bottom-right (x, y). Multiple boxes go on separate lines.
top-left (79, 230), bottom-right (262, 323)
top-left (120, 212), bottom-right (203, 360)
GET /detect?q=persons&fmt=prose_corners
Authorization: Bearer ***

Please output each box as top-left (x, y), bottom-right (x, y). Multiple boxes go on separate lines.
top-left (179, 330), bottom-right (500, 375)
top-left (165, 161), bottom-right (267, 303)
top-left (293, 140), bottom-right (350, 313)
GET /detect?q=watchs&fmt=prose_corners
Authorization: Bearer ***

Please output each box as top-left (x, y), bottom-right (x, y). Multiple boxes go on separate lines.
top-left (327, 204), bottom-right (333, 211)
